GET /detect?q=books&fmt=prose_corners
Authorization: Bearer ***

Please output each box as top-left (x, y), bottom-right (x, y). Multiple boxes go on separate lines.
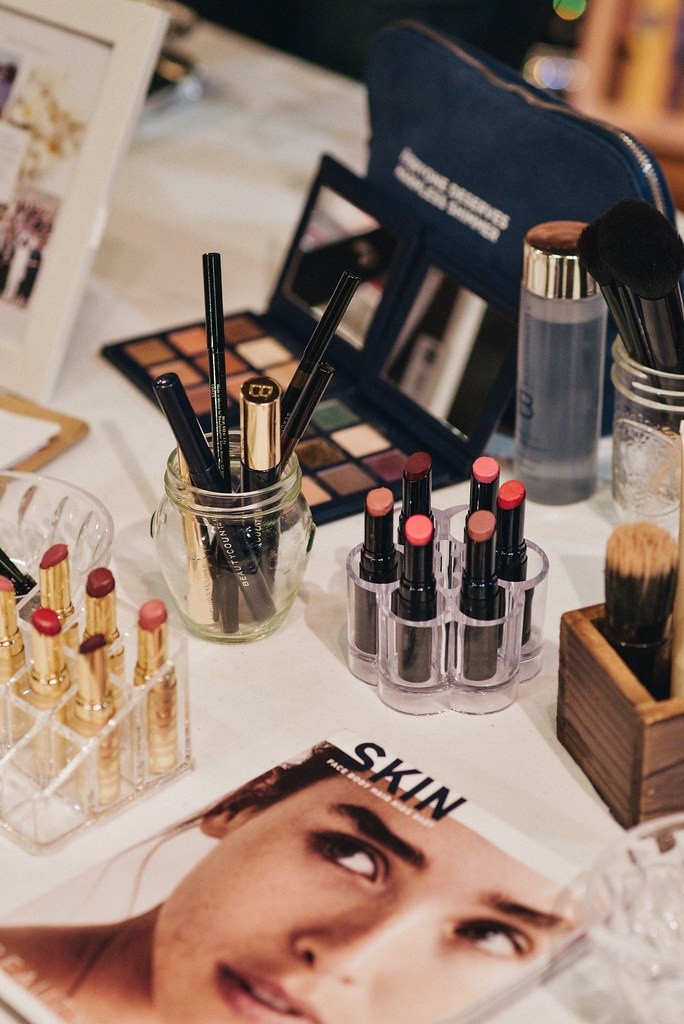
top-left (0, 721), bottom-right (615, 1024)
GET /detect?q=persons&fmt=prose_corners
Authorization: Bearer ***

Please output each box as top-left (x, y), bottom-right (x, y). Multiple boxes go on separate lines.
top-left (0, 743), bottom-right (573, 1024)
